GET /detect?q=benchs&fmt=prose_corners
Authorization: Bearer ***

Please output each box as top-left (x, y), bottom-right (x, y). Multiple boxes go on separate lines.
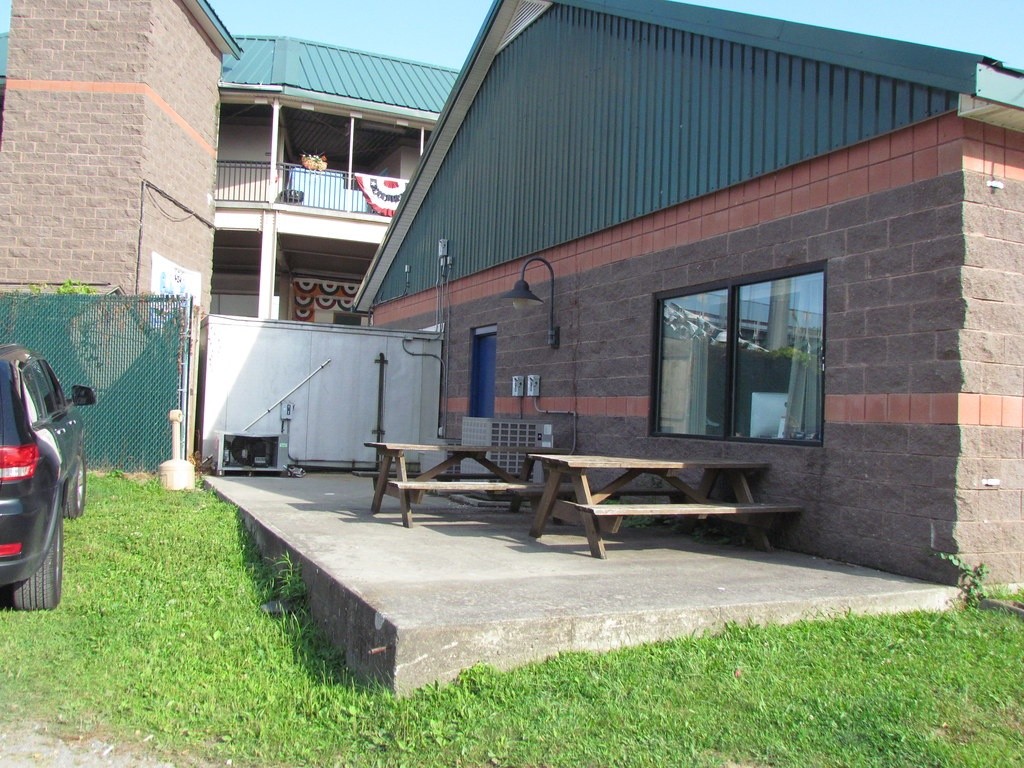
top-left (351, 470), bottom-right (531, 493)
top-left (392, 480), bottom-right (583, 530)
top-left (505, 485), bottom-right (710, 517)
top-left (575, 501), bottom-right (803, 560)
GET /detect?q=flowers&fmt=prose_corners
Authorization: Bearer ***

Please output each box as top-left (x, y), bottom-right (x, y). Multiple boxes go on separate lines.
top-left (301, 154), bottom-right (327, 170)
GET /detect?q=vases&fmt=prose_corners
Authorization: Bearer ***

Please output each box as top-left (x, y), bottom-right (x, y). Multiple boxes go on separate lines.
top-left (303, 161), bottom-right (327, 170)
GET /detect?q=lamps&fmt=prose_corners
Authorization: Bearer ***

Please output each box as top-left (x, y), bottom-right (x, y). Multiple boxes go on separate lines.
top-left (498, 257), bottom-right (561, 349)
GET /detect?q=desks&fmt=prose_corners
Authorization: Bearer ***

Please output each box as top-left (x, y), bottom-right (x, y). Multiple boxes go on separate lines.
top-left (527, 451), bottom-right (770, 559)
top-left (364, 442), bottom-right (572, 529)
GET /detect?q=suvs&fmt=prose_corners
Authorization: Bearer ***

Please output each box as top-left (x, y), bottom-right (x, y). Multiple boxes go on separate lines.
top-left (0, 340), bottom-right (98, 613)
